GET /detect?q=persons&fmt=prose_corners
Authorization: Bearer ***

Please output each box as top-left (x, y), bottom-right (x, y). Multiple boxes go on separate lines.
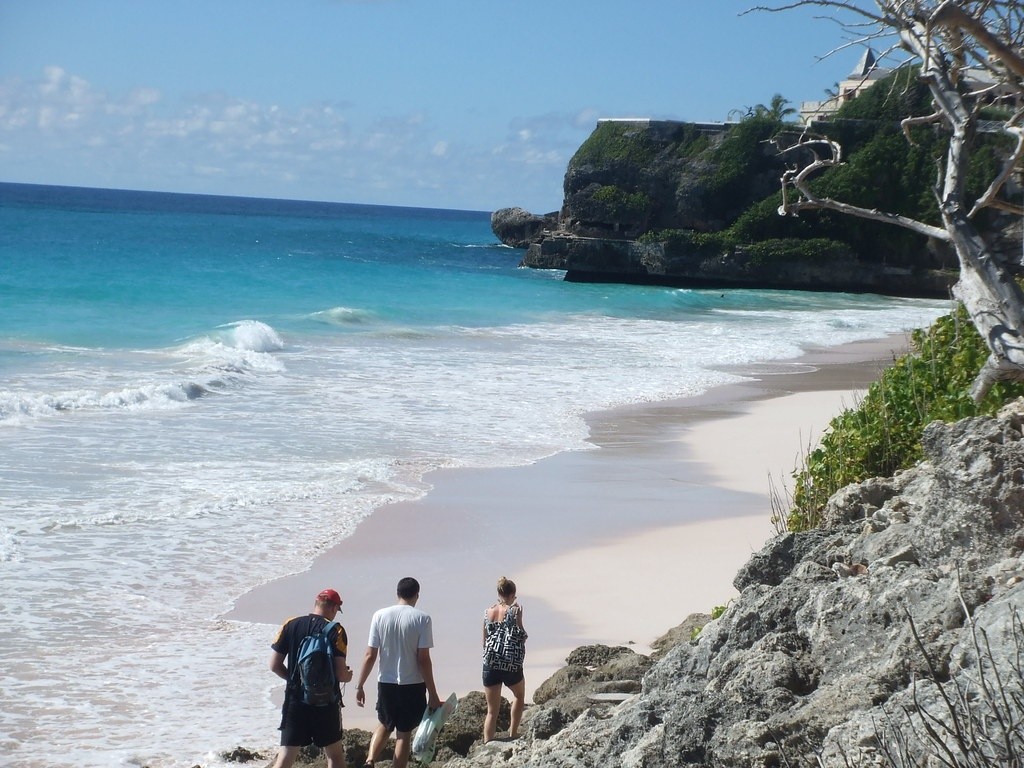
top-left (355, 577), bottom-right (443, 768)
top-left (270, 589), bottom-right (353, 768)
top-left (482, 577), bottom-right (527, 744)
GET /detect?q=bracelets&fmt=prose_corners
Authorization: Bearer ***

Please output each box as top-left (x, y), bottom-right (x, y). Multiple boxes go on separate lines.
top-left (355, 685), bottom-right (363, 689)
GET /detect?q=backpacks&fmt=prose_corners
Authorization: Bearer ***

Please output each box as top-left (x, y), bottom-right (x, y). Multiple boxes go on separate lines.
top-left (288, 622), bottom-right (345, 707)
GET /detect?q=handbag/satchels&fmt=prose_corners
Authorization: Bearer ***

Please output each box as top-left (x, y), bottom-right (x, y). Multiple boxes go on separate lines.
top-left (413, 705), bottom-right (437, 763)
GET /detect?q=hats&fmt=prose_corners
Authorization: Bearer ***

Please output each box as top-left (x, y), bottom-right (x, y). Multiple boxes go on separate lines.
top-left (313, 587), bottom-right (344, 615)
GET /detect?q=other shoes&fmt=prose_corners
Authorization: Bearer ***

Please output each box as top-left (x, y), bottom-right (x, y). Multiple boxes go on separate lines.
top-left (360, 759), bottom-right (374, 768)
